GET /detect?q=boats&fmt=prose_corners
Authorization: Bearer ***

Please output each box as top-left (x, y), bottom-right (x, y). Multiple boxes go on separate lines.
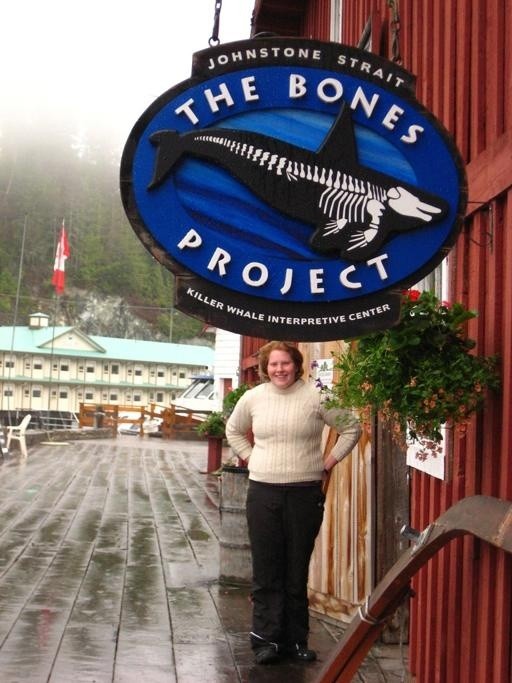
top-left (117, 375), bottom-right (216, 435)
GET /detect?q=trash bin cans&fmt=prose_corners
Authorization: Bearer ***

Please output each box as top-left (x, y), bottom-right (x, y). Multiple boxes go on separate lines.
top-left (220, 466), bottom-right (249, 513)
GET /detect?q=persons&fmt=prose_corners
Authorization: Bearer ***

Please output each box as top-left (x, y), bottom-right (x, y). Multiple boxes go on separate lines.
top-left (225, 341), bottom-right (363, 663)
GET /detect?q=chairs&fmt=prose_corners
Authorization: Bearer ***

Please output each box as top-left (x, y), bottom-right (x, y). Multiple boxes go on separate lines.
top-left (5, 412), bottom-right (32, 457)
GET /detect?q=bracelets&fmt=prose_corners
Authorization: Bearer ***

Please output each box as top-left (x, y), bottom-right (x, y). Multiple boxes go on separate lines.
top-left (324, 465), bottom-right (330, 477)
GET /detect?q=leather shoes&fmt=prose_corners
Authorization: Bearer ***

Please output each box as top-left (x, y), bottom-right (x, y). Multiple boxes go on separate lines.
top-left (252, 646), bottom-right (283, 665)
top-left (281, 643), bottom-right (316, 663)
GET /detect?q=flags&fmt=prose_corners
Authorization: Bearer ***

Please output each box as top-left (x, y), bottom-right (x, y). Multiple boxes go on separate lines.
top-left (51, 222), bottom-right (71, 295)
